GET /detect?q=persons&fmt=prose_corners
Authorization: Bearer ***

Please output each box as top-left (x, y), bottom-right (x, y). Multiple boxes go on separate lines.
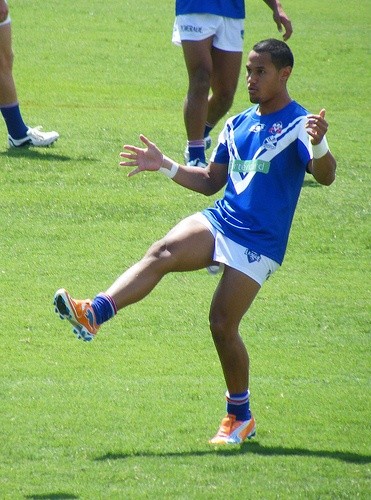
top-left (0, 0), bottom-right (60, 148)
top-left (175, 0), bottom-right (293, 168)
top-left (53, 38), bottom-right (336, 442)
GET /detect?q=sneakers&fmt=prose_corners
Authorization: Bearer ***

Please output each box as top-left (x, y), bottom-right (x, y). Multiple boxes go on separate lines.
top-left (53, 287), bottom-right (101, 342)
top-left (8, 127), bottom-right (60, 149)
top-left (210, 414), bottom-right (257, 453)
top-left (184, 138), bottom-right (211, 169)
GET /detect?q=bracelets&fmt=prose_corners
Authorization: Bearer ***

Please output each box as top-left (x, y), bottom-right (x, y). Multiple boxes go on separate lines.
top-left (159, 154), bottom-right (178, 178)
top-left (312, 135), bottom-right (329, 159)
